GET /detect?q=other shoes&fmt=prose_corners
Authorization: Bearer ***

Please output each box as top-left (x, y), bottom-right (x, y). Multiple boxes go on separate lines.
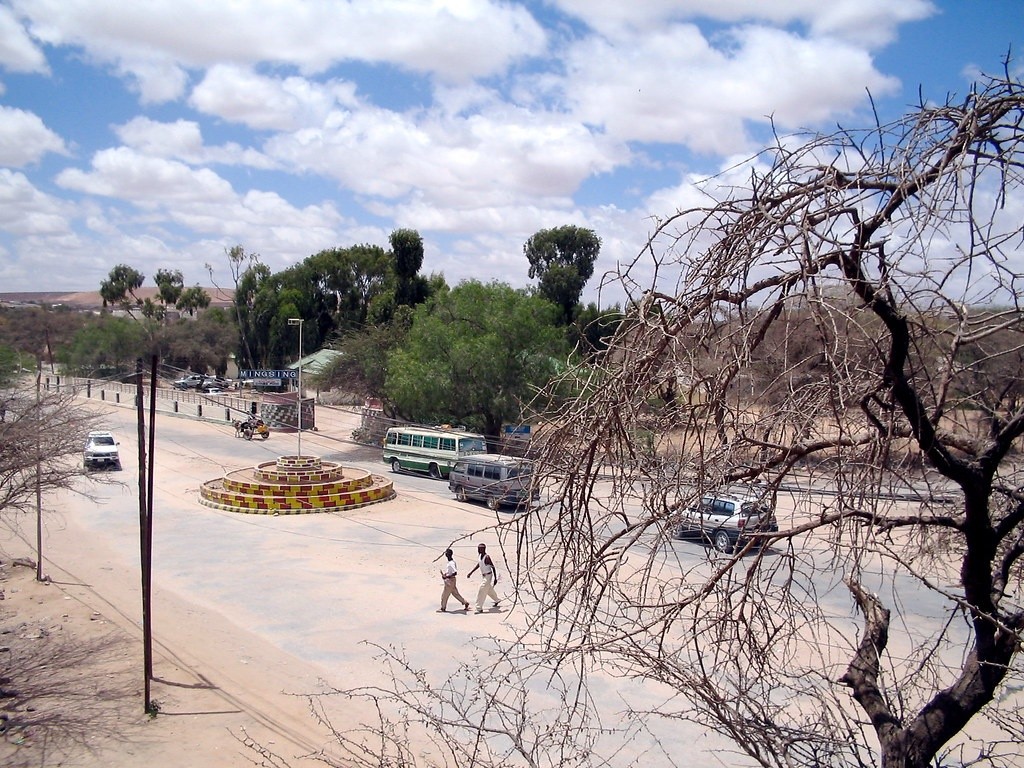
top-left (436, 608), bottom-right (445, 612)
top-left (474, 609), bottom-right (483, 614)
top-left (493, 601), bottom-right (501, 607)
top-left (464, 603), bottom-right (469, 610)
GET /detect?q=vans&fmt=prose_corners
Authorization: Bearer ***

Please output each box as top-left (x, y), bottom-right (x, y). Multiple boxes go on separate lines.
top-left (448, 453), bottom-right (540, 511)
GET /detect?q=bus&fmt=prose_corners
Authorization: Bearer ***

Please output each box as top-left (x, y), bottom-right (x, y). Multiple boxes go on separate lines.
top-left (382, 427), bottom-right (487, 479)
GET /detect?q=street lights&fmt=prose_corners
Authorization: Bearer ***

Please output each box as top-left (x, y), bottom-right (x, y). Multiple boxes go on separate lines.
top-left (288, 317), bottom-right (302, 459)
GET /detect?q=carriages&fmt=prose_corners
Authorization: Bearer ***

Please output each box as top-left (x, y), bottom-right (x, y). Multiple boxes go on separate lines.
top-left (232, 416), bottom-right (270, 441)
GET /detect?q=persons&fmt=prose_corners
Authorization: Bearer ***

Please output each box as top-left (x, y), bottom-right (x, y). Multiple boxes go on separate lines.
top-left (467, 543), bottom-right (501, 614)
top-left (436, 548), bottom-right (469, 612)
top-left (245, 411), bottom-right (253, 430)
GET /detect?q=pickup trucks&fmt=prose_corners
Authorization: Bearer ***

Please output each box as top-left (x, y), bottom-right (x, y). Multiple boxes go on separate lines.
top-left (173, 374), bottom-right (233, 390)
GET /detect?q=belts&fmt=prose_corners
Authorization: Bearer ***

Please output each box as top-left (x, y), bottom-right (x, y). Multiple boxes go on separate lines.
top-left (482, 572), bottom-right (492, 576)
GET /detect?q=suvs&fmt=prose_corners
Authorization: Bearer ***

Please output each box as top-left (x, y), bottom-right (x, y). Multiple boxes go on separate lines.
top-left (83, 431), bottom-right (120, 467)
top-left (669, 492), bottom-right (779, 554)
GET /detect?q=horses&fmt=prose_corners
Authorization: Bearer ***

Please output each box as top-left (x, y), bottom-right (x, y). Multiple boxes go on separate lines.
top-left (232, 418), bottom-right (242, 438)
